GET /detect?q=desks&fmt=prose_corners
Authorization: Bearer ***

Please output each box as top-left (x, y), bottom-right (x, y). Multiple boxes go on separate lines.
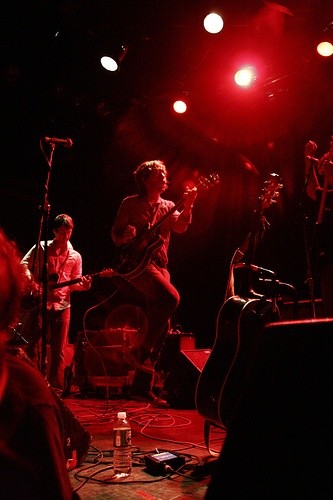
top-left (160, 332), bottom-right (198, 410)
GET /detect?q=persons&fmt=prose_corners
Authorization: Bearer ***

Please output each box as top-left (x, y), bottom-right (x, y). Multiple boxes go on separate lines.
top-left (111, 161), bottom-right (197, 400)
top-left (0, 229), bottom-right (75, 500)
top-left (21, 214), bottom-right (92, 398)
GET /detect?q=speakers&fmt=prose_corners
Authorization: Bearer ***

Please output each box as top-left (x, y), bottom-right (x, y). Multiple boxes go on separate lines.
top-left (159, 332), bottom-right (212, 410)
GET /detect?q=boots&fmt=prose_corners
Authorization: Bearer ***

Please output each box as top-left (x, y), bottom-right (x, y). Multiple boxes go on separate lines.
top-left (129, 365), bottom-right (165, 405)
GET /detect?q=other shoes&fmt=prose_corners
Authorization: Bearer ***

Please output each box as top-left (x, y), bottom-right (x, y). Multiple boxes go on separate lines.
top-left (55, 385), bottom-right (63, 391)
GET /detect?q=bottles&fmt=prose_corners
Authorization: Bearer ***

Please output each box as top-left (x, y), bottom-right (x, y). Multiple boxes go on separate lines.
top-left (112, 412), bottom-right (132, 478)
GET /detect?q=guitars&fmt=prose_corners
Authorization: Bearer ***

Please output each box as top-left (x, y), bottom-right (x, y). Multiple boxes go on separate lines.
top-left (194, 173), bottom-right (283, 430)
top-left (19, 268), bottom-right (114, 310)
top-left (112, 171), bottom-right (219, 280)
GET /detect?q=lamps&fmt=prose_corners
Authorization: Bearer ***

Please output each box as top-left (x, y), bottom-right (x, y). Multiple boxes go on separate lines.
top-left (100, 36), bottom-right (128, 73)
top-left (172, 87), bottom-right (192, 114)
top-left (315, 19), bottom-right (333, 58)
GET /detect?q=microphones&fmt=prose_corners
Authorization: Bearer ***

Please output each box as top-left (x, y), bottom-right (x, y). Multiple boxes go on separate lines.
top-left (43, 136), bottom-right (73, 148)
